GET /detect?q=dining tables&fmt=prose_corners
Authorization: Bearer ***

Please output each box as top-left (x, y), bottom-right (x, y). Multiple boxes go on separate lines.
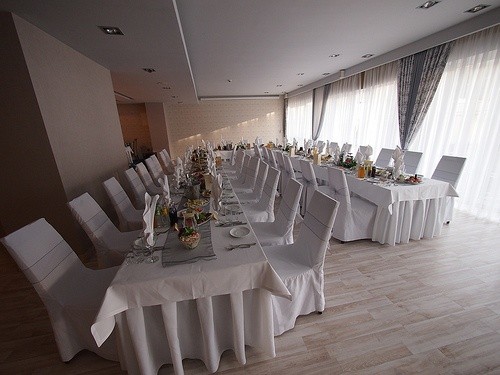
top-left (89, 136), bottom-right (292, 375)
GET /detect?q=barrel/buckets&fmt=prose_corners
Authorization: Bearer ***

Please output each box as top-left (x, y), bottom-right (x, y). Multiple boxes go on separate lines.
top-left (183, 181), bottom-right (201, 200)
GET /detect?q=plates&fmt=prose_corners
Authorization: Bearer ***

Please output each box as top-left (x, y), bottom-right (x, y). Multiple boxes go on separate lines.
top-left (176, 198), bottom-right (213, 228)
top-left (229, 226), bottom-right (250, 238)
top-left (135, 235), bottom-right (156, 248)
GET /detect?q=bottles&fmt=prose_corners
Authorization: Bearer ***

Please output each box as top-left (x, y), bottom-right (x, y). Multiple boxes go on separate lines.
top-left (358, 164), bottom-right (376, 178)
top-left (155, 201), bottom-right (177, 229)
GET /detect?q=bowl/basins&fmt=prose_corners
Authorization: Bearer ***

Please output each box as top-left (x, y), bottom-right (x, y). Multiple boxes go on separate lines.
top-left (178, 234), bottom-right (201, 249)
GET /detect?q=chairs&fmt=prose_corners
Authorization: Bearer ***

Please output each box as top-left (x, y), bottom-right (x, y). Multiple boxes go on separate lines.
top-left (1, 147), bottom-right (175, 363)
top-left (218, 137), bottom-right (467, 339)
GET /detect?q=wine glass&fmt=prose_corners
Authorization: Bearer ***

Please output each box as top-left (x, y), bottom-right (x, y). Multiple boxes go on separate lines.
top-left (144, 238), bottom-right (159, 263)
top-left (391, 170), bottom-right (401, 186)
